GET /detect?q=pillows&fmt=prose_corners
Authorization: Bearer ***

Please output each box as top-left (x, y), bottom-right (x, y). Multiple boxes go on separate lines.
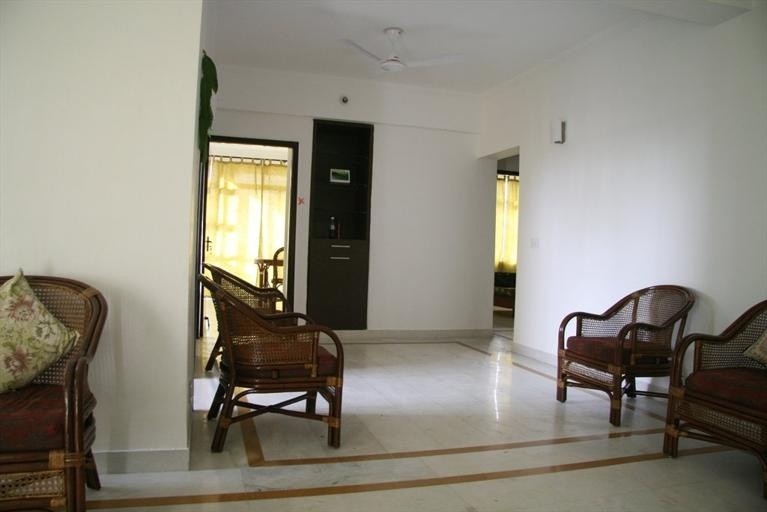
top-left (0, 266), bottom-right (82, 396)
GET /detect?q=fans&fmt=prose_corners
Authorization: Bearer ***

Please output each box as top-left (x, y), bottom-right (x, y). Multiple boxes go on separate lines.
top-left (339, 24), bottom-right (456, 80)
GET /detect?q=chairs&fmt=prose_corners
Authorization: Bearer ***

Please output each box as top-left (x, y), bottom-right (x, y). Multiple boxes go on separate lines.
top-left (555, 285), bottom-right (696, 426)
top-left (197, 248), bottom-right (343, 455)
top-left (664, 300), bottom-right (767, 495)
top-left (0, 273), bottom-right (108, 512)
